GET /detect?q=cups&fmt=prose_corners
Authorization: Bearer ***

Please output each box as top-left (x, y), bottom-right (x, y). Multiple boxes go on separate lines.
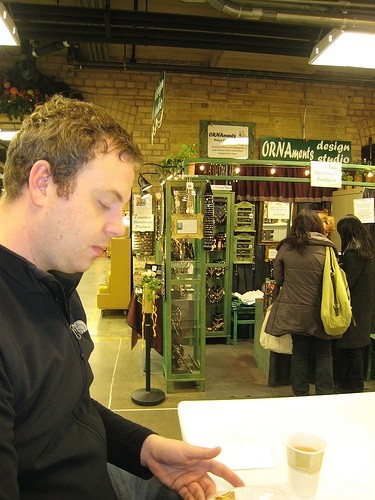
top-left (286, 433), bottom-right (327, 500)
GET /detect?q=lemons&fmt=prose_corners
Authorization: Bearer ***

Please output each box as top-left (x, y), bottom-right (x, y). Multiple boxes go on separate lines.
top-left (27, 89), bottom-right (34, 95)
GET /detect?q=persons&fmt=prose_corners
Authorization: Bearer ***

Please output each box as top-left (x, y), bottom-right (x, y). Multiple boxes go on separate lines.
top-left (0, 92), bottom-right (245, 500)
top-left (264, 209), bottom-right (375, 395)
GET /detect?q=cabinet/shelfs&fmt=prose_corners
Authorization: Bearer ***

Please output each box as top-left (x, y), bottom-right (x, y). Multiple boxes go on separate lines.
top-left (161, 179), bottom-right (235, 396)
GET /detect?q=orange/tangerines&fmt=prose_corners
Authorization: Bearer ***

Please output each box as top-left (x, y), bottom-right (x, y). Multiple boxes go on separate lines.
top-left (4, 81), bottom-right (18, 94)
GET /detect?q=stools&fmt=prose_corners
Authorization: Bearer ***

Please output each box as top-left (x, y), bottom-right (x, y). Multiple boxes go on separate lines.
top-left (231, 307), bottom-right (256, 351)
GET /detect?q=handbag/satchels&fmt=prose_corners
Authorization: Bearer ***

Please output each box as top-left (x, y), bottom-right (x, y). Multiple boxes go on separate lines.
top-left (321, 246), bottom-right (352, 335)
top-left (260, 283), bottom-right (293, 354)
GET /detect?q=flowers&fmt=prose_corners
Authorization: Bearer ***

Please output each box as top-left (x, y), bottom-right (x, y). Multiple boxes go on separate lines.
top-left (0, 72), bottom-right (87, 121)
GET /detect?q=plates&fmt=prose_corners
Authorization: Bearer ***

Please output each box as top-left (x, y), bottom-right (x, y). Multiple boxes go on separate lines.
top-left (206, 486), bottom-right (296, 500)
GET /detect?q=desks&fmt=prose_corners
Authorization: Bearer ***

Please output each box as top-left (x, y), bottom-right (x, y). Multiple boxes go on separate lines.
top-left (178, 392), bottom-right (375, 500)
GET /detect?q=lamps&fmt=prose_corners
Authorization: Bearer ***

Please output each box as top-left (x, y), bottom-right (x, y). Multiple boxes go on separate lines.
top-left (138, 172), bottom-right (159, 199)
top-left (0, 129), bottom-right (17, 140)
top-left (137, 163), bottom-right (178, 191)
top-left (0, 2), bottom-right (21, 47)
top-left (308, 28), bottom-right (375, 68)
